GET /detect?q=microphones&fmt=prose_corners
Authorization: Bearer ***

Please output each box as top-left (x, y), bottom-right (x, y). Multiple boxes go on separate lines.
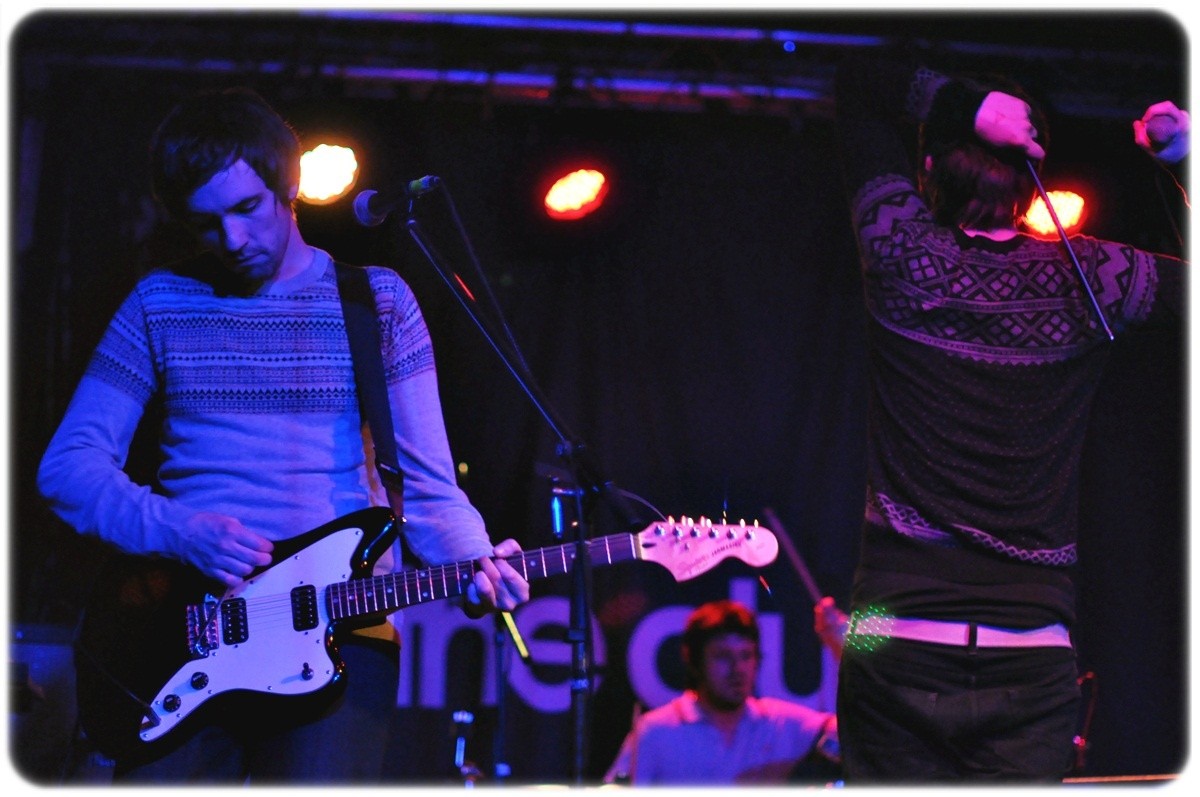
top-left (352, 175), bottom-right (442, 227)
top-left (1146, 116), bottom-right (1177, 145)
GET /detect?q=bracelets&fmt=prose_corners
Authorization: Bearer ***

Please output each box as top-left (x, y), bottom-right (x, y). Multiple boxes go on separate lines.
top-left (817, 738), bottom-right (840, 756)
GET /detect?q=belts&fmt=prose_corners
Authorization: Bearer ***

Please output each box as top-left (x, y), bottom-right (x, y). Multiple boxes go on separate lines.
top-left (849, 612), bottom-right (1072, 650)
top-left (354, 620), bottom-right (401, 649)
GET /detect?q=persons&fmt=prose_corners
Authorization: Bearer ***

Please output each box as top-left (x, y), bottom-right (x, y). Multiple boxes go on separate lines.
top-left (602, 601), bottom-right (837, 785)
top-left (37, 90), bottom-right (532, 787)
top-left (829, 57), bottom-right (1180, 780)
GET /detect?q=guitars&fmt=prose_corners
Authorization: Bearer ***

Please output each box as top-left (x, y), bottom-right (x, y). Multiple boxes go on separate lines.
top-left (75, 502), bottom-right (783, 764)
